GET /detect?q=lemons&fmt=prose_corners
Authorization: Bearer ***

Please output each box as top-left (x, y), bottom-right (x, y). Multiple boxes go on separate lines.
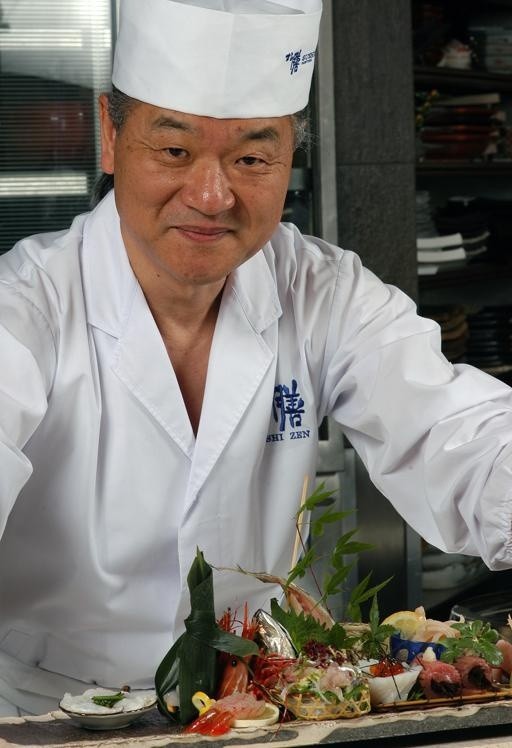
top-left (380, 610), bottom-right (420, 641)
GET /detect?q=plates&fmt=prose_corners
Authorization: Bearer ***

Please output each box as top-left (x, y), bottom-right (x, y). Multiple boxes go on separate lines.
top-left (414, 187), bottom-right (510, 372)
top-left (59, 688), bottom-right (158, 729)
top-left (416, 99), bottom-right (506, 159)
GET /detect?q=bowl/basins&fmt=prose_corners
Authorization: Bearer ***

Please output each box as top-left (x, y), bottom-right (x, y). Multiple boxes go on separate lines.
top-left (470, 23), bottom-right (512, 78)
top-left (355, 658), bottom-right (422, 703)
top-left (443, 48), bottom-right (472, 69)
top-left (228, 701), bottom-right (279, 730)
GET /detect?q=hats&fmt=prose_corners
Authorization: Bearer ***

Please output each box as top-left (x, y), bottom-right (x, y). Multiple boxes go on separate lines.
top-left (112, 0), bottom-right (323, 119)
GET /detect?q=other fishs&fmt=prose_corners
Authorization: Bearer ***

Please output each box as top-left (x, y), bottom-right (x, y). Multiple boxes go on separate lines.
top-left (249, 609), bottom-right (300, 663)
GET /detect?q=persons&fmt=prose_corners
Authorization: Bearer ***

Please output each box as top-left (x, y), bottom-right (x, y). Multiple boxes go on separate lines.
top-left (0, 2), bottom-right (512, 722)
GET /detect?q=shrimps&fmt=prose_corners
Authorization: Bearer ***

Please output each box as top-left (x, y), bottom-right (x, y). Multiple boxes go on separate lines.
top-left (182, 691), bottom-right (255, 733)
top-left (199, 698), bottom-right (266, 736)
top-left (217, 600), bottom-right (265, 700)
